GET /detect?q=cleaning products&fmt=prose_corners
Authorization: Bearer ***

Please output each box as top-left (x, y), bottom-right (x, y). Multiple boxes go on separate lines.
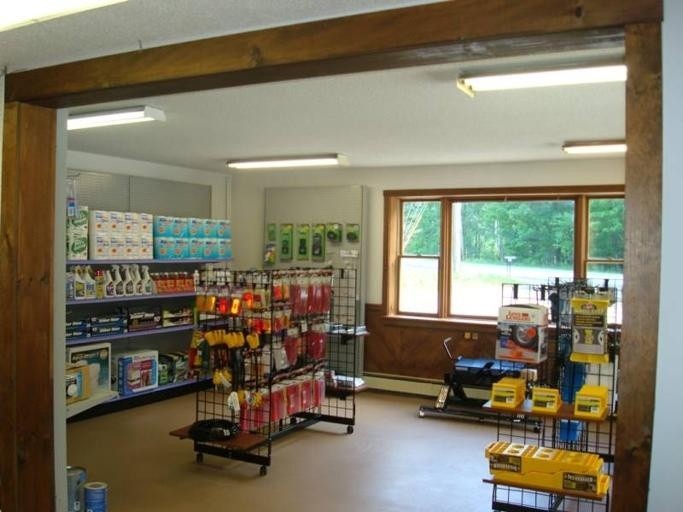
top-left (193, 268), bottom-right (232, 289)
top-left (66, 265), bottom-right (153, 300)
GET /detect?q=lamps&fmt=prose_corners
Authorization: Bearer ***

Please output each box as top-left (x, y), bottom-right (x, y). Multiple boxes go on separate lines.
top-left (224, 153), bottom-right (349, 169)
top-left (66, 104), bottom-right (166, 130)
top-left (562, 143), bottom-right (627, 153)
top-left (455, 64), bottom-right (627, 97)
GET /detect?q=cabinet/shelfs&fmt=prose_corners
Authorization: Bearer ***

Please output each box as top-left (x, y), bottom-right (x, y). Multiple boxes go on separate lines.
top-left (65, 257), bottom-right (233, 424)
